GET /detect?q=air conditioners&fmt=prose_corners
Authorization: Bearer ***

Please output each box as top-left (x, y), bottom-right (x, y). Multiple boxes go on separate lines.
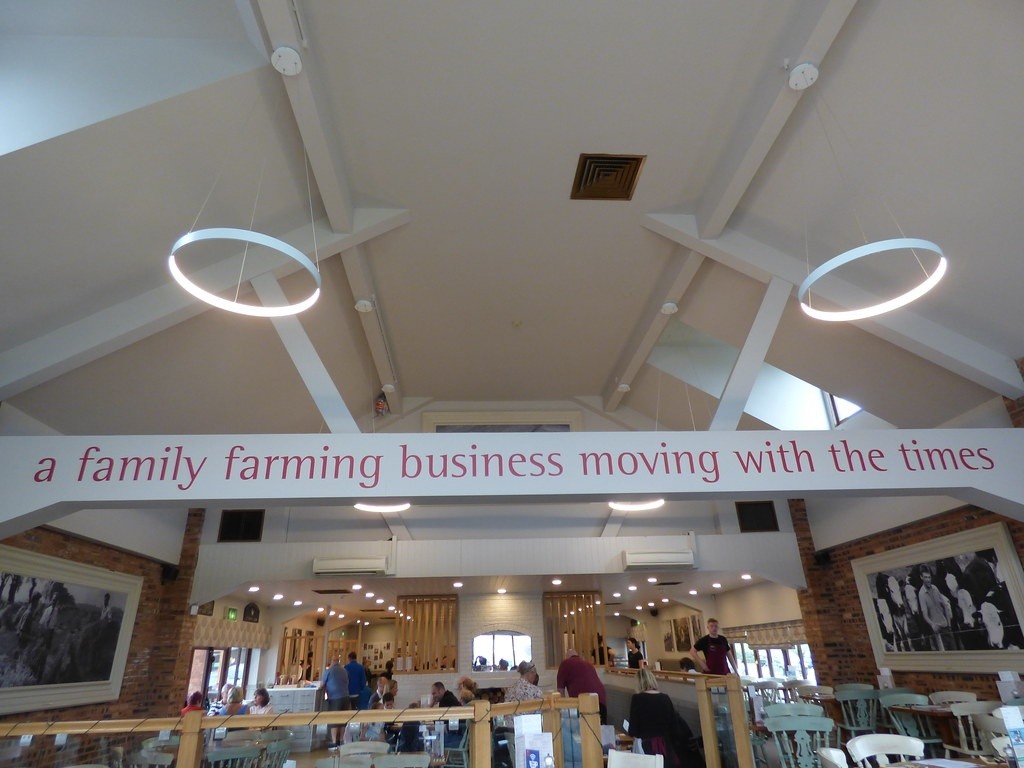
top-left (311, 555), bottom-right (387, 578)
top-left (621, 550), bottom-right (694, 570)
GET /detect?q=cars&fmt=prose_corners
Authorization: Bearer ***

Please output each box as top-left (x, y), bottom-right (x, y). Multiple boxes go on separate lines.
top-left (206, 683), bottom-right (220, 700)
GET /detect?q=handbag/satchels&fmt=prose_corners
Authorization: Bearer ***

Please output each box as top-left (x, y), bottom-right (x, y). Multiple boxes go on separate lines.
top-left (666, 694), bottom-right (693, 738)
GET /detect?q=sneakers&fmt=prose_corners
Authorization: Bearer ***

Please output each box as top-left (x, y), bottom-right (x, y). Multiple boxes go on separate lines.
top-left (328, 740), bottom-right (344, 750)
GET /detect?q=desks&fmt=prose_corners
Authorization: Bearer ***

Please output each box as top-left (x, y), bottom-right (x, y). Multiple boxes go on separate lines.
top-left (154, 734), bottom-right (270, 760)
top-left (889, 703), bottom-right (970, 757)
top-left (800, 694), bottom-right (862, 704)
top-left (346, 750), bottom-right (446, 768)
top-left (885, 757), bottom-right (1009, 768)
top-left (615, 727), bottom-right (634, 745)
top-left (383, 720), bottom-right (442, 753)
top-left (752, 721), bottom-right (837, 765)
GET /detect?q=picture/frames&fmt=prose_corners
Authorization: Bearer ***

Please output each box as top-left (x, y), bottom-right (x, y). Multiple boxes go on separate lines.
top-left (292, 627), bottom-right (303, 664)
top-left (849, 520), bottom-right (1024, 673)
top-left (0, 542), bottom-right (144, 717)
top-left (420, 409), bottom-right (584, 432)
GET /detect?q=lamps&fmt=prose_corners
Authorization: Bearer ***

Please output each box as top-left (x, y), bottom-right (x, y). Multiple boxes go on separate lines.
top-left (328, 608), bottom-right (336, 616)
top-left (338, 611), bottom-right (345, 620)
top-left (167, 50), bottom-right (323, 319)
top-left (608, 383), bottom-right (665, 512)
top-left (316, 607), bottom-right (325, 613)
top-left (363, 621), bottom-right (370, 626)
top-left (789, 62), bottom-right (950, 322)
top-left (353, 384), bottom-right (411, 513)
top-left (292, 599), bottom-right (303, 606)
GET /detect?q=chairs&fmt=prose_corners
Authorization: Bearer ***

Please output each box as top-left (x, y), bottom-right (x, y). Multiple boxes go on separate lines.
top-left (105, 672), bottom-right (1024, 768)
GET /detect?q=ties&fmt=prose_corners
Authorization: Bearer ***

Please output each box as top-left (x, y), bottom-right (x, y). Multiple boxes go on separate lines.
top-left (46, 607), bottom-right (54, 628)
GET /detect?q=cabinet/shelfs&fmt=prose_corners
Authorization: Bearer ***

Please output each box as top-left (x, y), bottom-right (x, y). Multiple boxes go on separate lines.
top-left (266, 688), bottom-right (323, 751)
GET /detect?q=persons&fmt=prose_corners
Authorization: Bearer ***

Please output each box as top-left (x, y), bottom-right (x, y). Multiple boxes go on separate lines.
top-left (679, 657), bottom-right (705, 684)
top-left (297, 651), bottom-right (319, 686)
top-left (919, 566), bottom-right (958, 651)
top-left (414, 656), bottom-right (455, 671)
top-left (627, 638), bottom-right (643, 674)
top-left (504, 660), bottom-right (544, 768)
top-left (689, 618), bottom-right (739, 677)
top-left (557, 649), bottom-right (607, 725)
top-left (629, 668), bottom-right (684, 768)
top-left (361, 661), bottom-right (394, 683)
top-left (499, 659), bottom-right (509, 671)
top-left (430, 678), bottom-right (477, 748)
top-left (100, 593), bottom-right (112, 623)
top-left (344, 652), bottom-right (366, 710)
top-left (359, 677), bottom-right (421, 753)
top-left (321, 657), bottom-right (351, 750)
top-left (591, 638), bottom-right (615, 665)
top-left (16, 591), bottom-right (82, 684)
top-left (473, 656), bottom-right (492, 672)
top-left (181, 684), bottom-right (274, 768)
